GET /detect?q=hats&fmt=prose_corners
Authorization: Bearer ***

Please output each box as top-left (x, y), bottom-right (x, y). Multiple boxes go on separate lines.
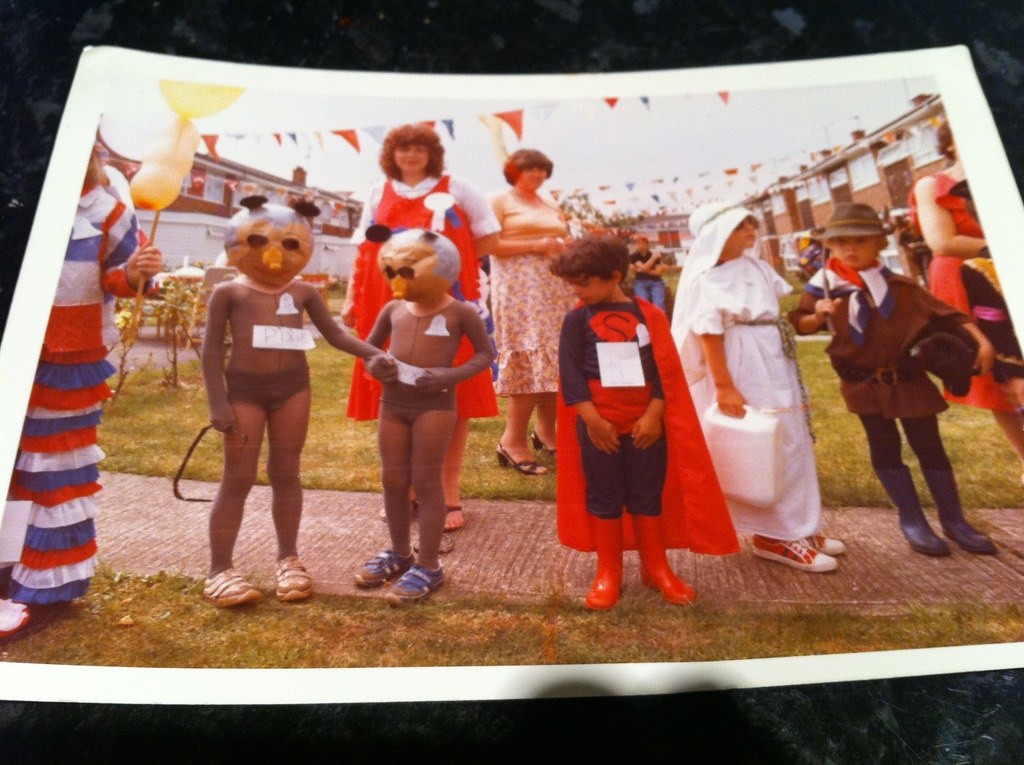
top-left (811, 203), bottom-right (884, 239)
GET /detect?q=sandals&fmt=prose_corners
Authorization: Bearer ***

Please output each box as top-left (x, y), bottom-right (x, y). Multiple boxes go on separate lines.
top-left (443, 505), bottom-right (463, 532)
top-left (379, 498), bottom-right (419, 522)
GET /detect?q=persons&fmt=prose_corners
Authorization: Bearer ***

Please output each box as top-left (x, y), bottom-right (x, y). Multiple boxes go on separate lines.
top-left (6, 146), bottom-right (162, 606)
top-left (355, 223), bottom-right (497, 603)
top-left (670, 202), bottom-right (844, 572)
top-left (787, 202), bottom-right (996, 555)
top-left (908, 119), bottom-right (1024, 460)
top-left (549, 232), bottom-right (740, 609)
top-left (629, 236), bottom-right (665, 309)
top-left (489, 149), bottom-right (573, 475)
top-left (341, 124), bottom-right (500, 531)
top-left (203, 196), bottom-right (388, 608)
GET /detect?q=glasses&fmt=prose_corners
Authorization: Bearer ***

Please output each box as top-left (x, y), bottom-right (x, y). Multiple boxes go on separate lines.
top-left (736, 215), bottom-right (759, 228)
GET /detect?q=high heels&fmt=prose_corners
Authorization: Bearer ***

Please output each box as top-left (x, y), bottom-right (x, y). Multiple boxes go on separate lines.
top-left (496, 443), bottom-right (549, 475)
top-left (532, 432), bottom-right (556, 455)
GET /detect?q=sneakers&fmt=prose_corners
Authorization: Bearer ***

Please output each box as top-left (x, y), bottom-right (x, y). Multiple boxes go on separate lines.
top-left (798, 534), bottom-right (845, 556)
top-left (353, 543), bottom-right (415, 587)
top-left (753, 535), bottom-right (838, 573)
top-left (202, 566), bottom-right (261, 607)
top-left (384, 559), bottom-right (445, 604)
top-left (274, 554), bottom-right (314, 602)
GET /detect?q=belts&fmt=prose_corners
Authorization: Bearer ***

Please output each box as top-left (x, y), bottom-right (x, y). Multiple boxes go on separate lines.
top-left (843, 366), bottom-right (914, 384)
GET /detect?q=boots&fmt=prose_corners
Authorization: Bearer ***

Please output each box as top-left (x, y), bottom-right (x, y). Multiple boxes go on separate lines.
top-left (873, 464), bottom-right (951, 557)
top-left (922, 459), bottom-right (999, 555)
top-left (586, 513), bottom-right (622, 611)
top-left (629, 512), bottom-right (696, 606)
